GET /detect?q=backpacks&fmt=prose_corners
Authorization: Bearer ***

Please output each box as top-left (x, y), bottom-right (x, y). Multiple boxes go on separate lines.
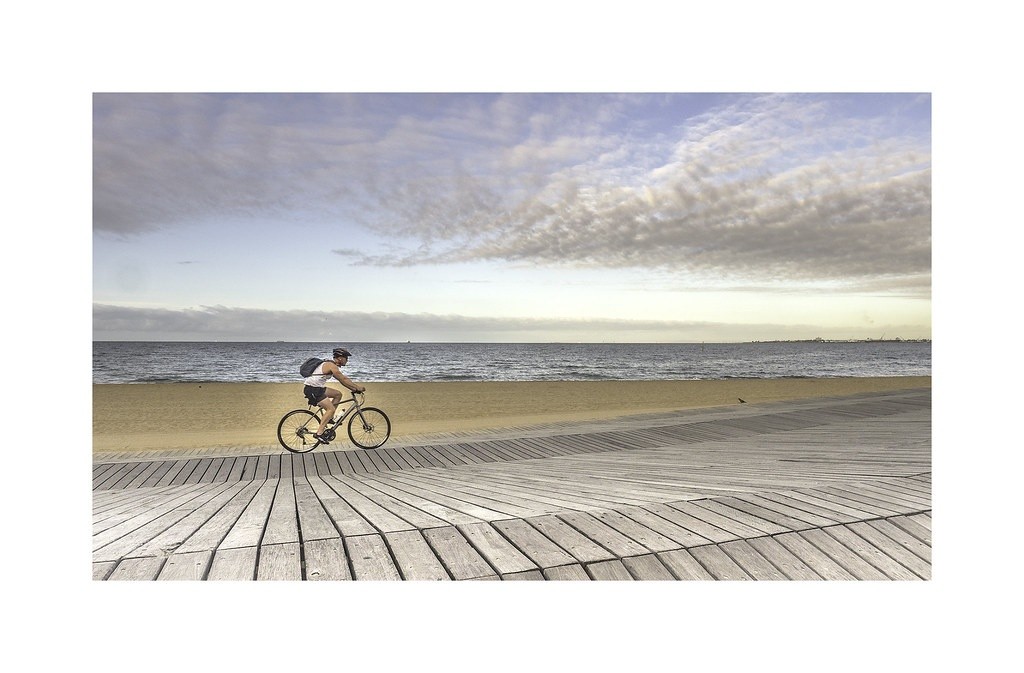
top-left (300, 357), bottom-right (336, 378)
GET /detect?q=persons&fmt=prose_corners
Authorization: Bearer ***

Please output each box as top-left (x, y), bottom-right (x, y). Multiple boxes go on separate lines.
top-left (303, 348), bottom-right (365, 445)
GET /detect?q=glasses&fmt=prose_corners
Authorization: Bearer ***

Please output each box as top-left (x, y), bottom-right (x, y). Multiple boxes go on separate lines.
top-left (343, 356), bottom-right (348, 360)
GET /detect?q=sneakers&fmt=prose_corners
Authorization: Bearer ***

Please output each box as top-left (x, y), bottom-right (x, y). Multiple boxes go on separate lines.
top-left (328, 419), bottom-right (342, 425)
top-left (313, 433), bottom-right (330, 444)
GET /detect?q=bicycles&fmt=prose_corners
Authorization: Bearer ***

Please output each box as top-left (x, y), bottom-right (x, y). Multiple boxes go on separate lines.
top-left (276, 386), bottom-right (391, 454)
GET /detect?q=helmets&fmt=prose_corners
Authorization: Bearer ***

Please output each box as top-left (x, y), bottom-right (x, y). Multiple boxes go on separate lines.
top-left (333, 348), bottom-right (352, 357)
